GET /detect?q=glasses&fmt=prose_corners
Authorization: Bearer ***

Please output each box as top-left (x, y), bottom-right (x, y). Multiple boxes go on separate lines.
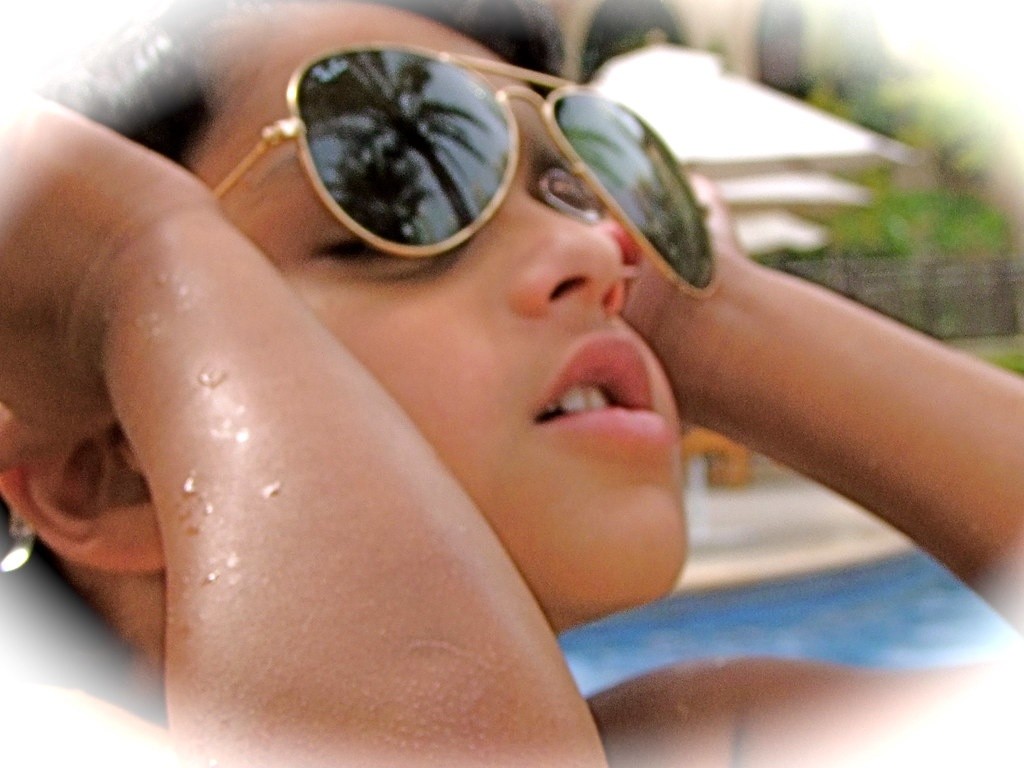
top-left (213, 42), bottom-right (724, 299)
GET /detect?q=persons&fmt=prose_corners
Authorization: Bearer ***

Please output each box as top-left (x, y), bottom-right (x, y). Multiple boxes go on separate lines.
top-left (0, 0), bottom-right (1024, 768)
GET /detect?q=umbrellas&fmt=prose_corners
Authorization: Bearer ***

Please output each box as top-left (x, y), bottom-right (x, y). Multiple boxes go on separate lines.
top-left (591, 45), bottom-right (918, 257)
